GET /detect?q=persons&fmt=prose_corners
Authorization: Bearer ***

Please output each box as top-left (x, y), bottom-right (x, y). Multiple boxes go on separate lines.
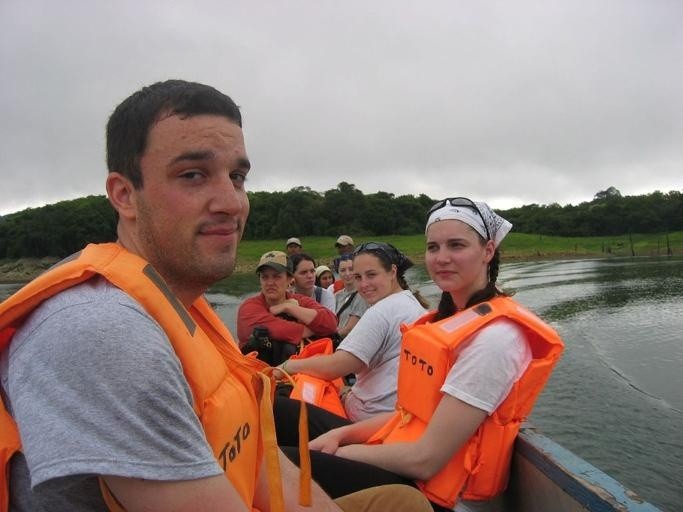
top-left (1, 80), bottom-right (436, 512)
top-left (234, 235), bottom-right (369, 364)
top-left (262, 240), bottom-right (429, 426)
top-left (262, 197), bottom-right (565, 512)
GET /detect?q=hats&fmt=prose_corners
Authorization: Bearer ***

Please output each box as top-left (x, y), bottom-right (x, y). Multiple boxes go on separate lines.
top-left (287, 237), bottom-right (302, 248)
top-left (315, 265), bottom-right (331, 278)
top-left (334, 235), bottom-right (354, 247)
top-left (255, 251), bottom-right (293, 276)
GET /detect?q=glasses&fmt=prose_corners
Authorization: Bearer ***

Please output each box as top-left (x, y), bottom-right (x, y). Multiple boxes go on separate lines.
top-left (333, 254), bottom-right (349, 266)
top-left (352, 242), bottom-right (393, 262)
top-left (424, 198), bottom-right (490, 240)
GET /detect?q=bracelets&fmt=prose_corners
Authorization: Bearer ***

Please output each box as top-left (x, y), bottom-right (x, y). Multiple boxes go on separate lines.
top-left (281, 360), bottom-right (290, 375)
